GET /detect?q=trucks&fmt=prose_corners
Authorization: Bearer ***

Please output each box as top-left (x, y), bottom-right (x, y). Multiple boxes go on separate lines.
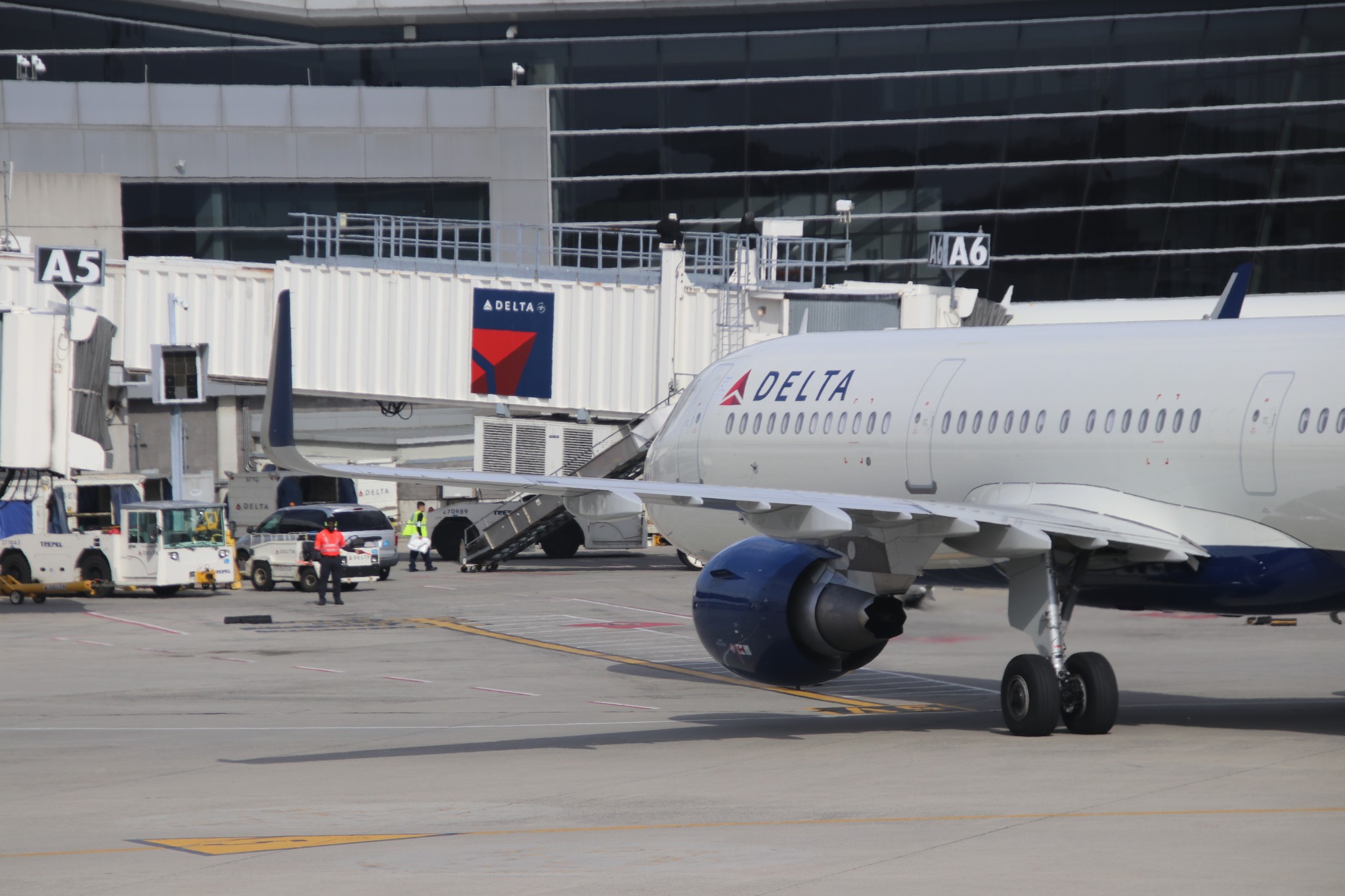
top-left (1, 472), bottom-right (238, 598)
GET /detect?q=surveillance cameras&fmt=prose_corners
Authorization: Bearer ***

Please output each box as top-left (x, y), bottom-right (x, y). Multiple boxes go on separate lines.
top-left (517, 68), bottom-right (524, 75)
top-left (21, 60), bottom-right (30, 67)
top-left (506, 30), bottom-right (515, 40)
top-left (36, 63), bottom-right (46, 73)
top-left (182, 303), bottom-right (188, 311)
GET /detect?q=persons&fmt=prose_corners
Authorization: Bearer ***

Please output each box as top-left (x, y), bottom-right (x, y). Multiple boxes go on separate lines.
top-left (401, 502), bottom-right (437, 572)
top-left (309, 517), bottom-right (365, 605)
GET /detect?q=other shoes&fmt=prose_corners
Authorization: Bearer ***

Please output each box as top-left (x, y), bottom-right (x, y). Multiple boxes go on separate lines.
top-left (408, 566), bottom-right (419, 572)
top-left (425, 566), bottom-right (437, 572)
top-left (319, 599), bottom-right (327, 606)
top-left (334, 598), bottom-right (344, 605)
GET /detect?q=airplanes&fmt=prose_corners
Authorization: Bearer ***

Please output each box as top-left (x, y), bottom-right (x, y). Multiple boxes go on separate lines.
top-left (259, 275), bottom-right (1345, 736)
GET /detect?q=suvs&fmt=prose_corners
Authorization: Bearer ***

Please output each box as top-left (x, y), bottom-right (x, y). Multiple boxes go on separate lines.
top-left (236, 503), bottom-right (399, 593)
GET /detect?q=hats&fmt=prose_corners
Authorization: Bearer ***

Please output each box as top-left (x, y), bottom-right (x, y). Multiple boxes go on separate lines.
top-left (326, 516), bottom-right (336, 524)
